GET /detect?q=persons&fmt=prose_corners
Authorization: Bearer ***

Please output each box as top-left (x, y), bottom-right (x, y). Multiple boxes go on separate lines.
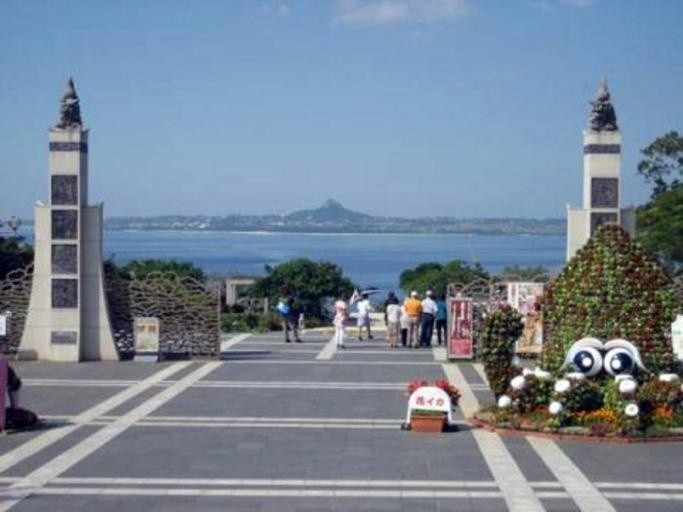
top-left (331, 290), bottom-right (348, 350)
top-left (277, 291), bottom-right (303, 343)
top-left (355, 291), bottom-right (374, 340)
top-left (381, 289), bottom-right (447, 347)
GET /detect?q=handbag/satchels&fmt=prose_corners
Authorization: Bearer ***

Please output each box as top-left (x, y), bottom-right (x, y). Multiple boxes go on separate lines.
top-left (276, 302), bottom-right (292, 317)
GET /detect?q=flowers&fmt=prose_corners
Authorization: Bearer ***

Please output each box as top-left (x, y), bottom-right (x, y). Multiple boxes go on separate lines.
top-left (403, 380), bottom-right (461, 415)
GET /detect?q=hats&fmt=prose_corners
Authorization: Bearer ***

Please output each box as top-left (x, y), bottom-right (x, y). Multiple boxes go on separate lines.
top-left (411, 291), bottom-right (419, 296)
top-left (427, 290), bottom-right (432, 295)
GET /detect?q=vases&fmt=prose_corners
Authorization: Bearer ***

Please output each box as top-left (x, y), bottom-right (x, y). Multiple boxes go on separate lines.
top-left (411, 415), bottom-right (446, 432)
